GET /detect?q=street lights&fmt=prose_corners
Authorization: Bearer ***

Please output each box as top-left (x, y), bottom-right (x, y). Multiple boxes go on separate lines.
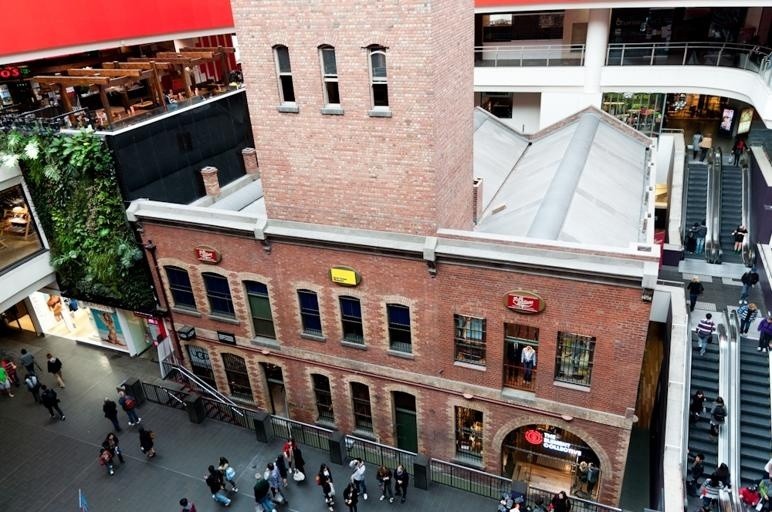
top-left (293, 469), bottom-right (304, 480)
top-left (316, 476), bottom-right (320, 485)
top-left (755, 504), bottom-right (763, 511)
top-left (707, 335), bottom-right (712, 344)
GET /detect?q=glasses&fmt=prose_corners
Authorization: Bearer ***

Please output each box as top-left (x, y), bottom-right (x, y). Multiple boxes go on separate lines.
top-left (178, 326), bottom-right (195, 341)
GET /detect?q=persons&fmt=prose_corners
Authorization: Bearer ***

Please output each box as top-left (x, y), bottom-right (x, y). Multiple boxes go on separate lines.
top-left (0, 348), bottom-right (66, 421)
top-left (144, 332), bottom-right (157, 362)
top-left (569, 341), bottom-right (586, 372)
top-left (686, 447), bottom-right (772, 512)
top-left (98, 390), bottom-right (157, 476)
top-left (95, 310), bottom-right (124, 344)
top-left (178, 437), bottom-right (410, 512)
top-left (70, 68), bottom-right (244, 122)
top-left (47, 292), bottom-right (65, 324)
top-left (508, 338), bottom-right (535, 387)
top-left (692, 129), bottom-right (748, 167)
top-left (600, 105), bottom-right (649, 128)
top-left (692, 389), bottom-right (706, 428)
top-left (686, 220), bottom-right (772, 357)
top-left (710, 397), bottom-right (727, 436)
top-left (498, 459), bottom-right (599, 512)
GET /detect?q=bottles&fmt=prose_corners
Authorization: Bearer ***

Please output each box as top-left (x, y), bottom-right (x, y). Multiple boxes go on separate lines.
top-left (748, 303), bottom-right (757, 310)
top-left (255, 473), bottom-right (261, 479)
top-left (751, 268), bottom-right (757, 272)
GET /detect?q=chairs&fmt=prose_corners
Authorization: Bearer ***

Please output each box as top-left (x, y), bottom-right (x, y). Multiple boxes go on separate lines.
top-left (225, 499), bottom-right (231, 506)
top-left (698, 345), bottom-right (702, 347)
top-left (739, 300), bottom-right (748, 304)
top-left (757, 347), bottom-right (766, 352)
top-left (700, 352), bottom-right (703, 356)
top-left (380, 492), bottom-right (406, 503)
top-left (128, 418), bottom-right (141, 426)
top-left (364, 494), bottom-right (367, 501)
top-left (740, 332), bottom-right (746, 336)
top-left (231, 487), bottom-right (239, 492)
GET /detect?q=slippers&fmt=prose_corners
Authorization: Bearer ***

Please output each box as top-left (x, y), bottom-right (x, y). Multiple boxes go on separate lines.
top-left (226, 467), bottom-right (235, 480)
top-left (124, 396), bottom-right (135, 409)
top-left (714, 405), bottom-right (725, 421)
